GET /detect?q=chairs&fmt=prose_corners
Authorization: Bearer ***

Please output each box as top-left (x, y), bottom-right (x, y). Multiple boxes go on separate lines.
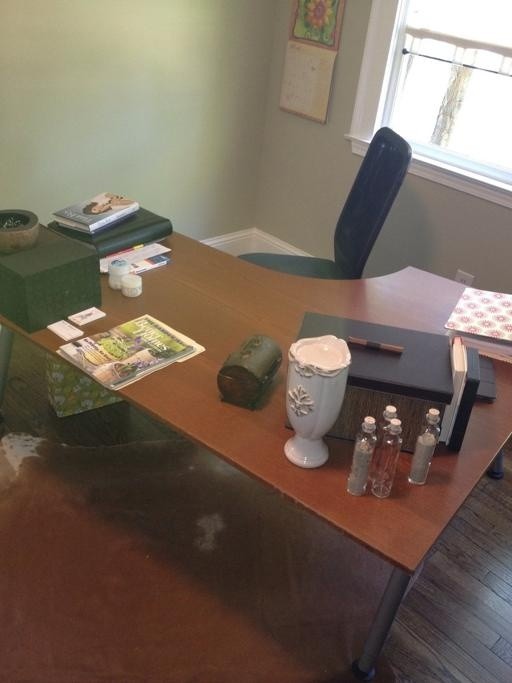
top-left (230, 122), bottom-right (410, 282)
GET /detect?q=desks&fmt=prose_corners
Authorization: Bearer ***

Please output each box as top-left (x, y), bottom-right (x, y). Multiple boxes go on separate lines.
top-left (2, 203), bottom-right (512, 683)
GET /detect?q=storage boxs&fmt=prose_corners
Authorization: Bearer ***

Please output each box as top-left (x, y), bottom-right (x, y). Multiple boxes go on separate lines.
top-left (0, 219), bottom-right (104, 336)
top-left (282, 303), bottom-right (457, 459)
top-left (39, 351), bottom-right (126, 419)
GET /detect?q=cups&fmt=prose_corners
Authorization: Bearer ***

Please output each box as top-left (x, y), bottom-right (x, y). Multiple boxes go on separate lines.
top-left (119, 273), bottom-right (143, 297)
top-left (281, 334), bottom-right (353, 468)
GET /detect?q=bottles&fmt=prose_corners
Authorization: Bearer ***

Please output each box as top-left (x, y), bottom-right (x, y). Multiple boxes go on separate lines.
top-left (405, 408), bottom-right (442, 485)
top-left (345, 405), bottom-right (401, 498)
top-left (106, 257), bottom-right (129, 291)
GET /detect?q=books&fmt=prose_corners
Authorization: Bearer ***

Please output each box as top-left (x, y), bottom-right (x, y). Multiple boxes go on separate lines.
top-left (55, 314), bottom-right (206, 390)
top-left (47, 191), bottom-right (173, 276)
top-left (434, 287), bottom-right (512, 453)
